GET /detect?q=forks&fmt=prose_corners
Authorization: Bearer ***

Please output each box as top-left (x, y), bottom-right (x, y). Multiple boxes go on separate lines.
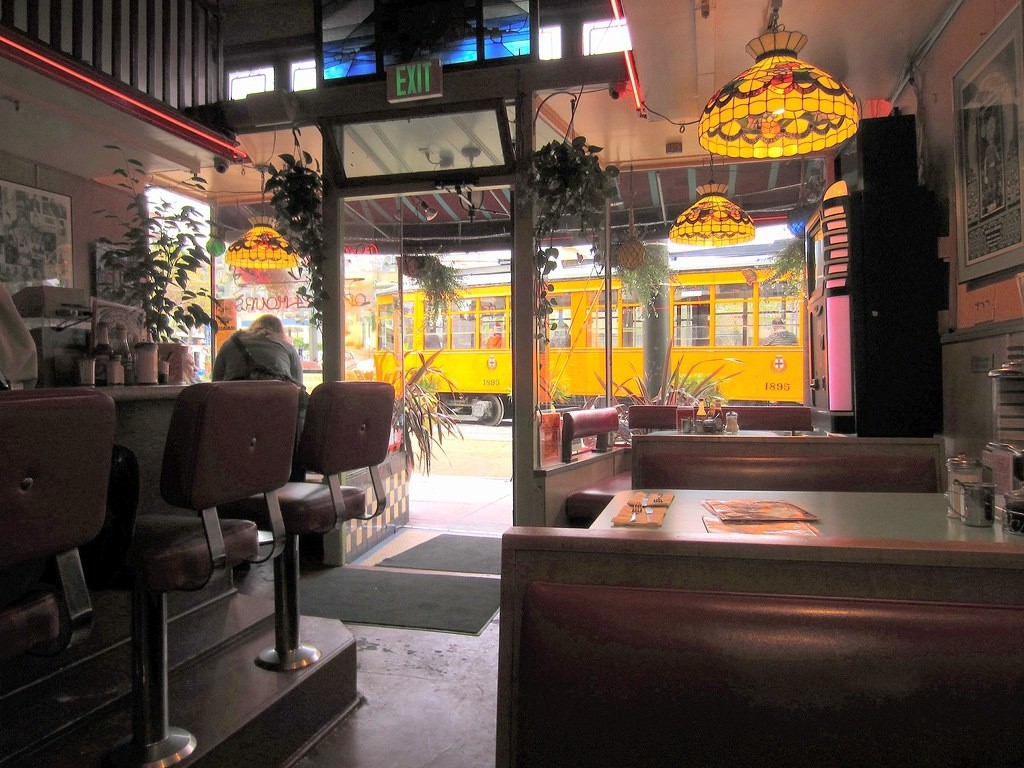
top-left (653, 489), bottom-right (666, 504)
top-left (629, 501), bottom-right (643, 522)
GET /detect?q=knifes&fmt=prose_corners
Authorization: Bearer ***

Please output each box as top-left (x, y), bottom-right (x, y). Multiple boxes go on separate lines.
top-left (642, 492), bottom-right (650, 507)
top-left (645, 508), bottom-right (654, 522)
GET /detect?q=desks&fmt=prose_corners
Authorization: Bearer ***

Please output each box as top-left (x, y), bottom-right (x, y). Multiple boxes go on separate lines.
top-left (584, 490), bottom-right (1013, 545)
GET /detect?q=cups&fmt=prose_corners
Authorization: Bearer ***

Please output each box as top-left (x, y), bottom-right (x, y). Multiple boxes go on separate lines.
top-left (681, 419), bottom-right (693, 433)
top-left (953, 478), bottom-right (995, 526)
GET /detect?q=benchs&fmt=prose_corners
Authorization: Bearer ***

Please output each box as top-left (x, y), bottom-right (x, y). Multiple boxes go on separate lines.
top-left (491, 523), bottom-right (1022, 768)
top-left (631, 435), bottom-right (951, 495)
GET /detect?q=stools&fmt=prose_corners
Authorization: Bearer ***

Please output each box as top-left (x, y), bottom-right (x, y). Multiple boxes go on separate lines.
top-left (0, 384), bottom-right (118, 676)
top-left (83, 379), bottom-right (306, 768)
top-left (199, 381), bottom-right (398, 670)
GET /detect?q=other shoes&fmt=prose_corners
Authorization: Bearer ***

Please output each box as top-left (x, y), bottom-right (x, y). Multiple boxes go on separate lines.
top-left (233, 561), bottom-right (251, 576)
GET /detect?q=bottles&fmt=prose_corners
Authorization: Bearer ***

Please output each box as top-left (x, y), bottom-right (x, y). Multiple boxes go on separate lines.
top-left (89, 320), bottom-right (114, 382)
top-left (944, 451), bottom-right (984, 518)
top-left (704, 417), bottom-right (716, 433)
top-left (695, 399), bottom-right (707, 433)
top-left (134, 340), bottom-right (161, 384)
top-left (106, 355), bottom-right (125, 386)
top-left (725, 412), bottom-right (739, 432)
top-left (77, 356), bottom-right (98, 385)
top-left (713, 399), bottom-right (723, 431)
top-left (116, 324), bottom-right (135, 385)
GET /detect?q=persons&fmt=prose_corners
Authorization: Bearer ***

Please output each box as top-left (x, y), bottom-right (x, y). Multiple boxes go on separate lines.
top-left (764, 318), bottom-right (798, 346)
top-left (213, 315), bottom-right (303, 577)
top-left (184, 354), bottom-right (197, 383)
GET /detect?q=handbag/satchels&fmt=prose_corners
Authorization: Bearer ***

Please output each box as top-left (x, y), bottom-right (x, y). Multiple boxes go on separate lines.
top-left (251, 364), bottom-right (311, 412)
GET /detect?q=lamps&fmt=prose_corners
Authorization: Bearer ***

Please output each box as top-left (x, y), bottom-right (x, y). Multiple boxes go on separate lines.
top-left (457, 147), bottom-right (488, 223)
top-left (414, 197), bottom-right (438, 222)
top-left (697, 28), bottom-right (864, 160)
top-left (224, 213), bottom-right (301, 272)
top-left (663, 147), bottom-right (759, 248)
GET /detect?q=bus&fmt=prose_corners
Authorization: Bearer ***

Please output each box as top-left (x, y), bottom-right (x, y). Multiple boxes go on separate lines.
top-left (374, 262), bottom-right (804, 425)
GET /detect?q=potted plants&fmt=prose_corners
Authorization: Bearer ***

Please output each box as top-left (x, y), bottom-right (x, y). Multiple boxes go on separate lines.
top-left (60, 143), bottom-right (221, 381)
